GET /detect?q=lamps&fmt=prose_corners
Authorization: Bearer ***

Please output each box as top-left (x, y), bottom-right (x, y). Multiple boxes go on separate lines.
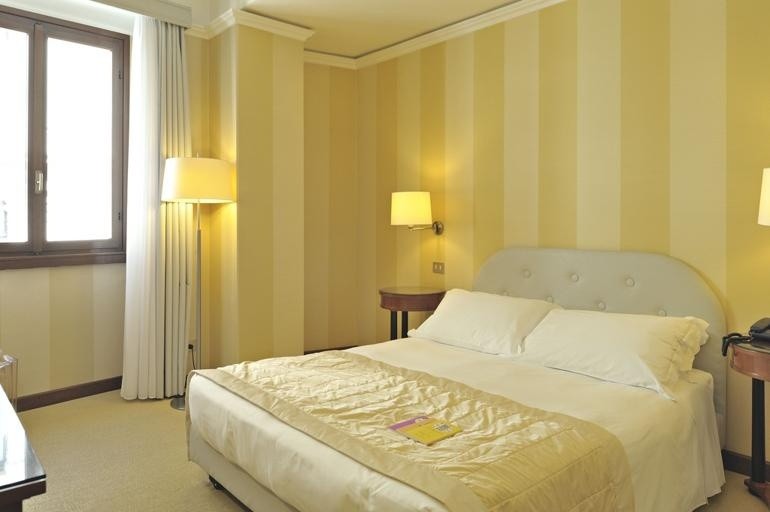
top-left (388, 190), bottom-right (442, 236)
top-left (161, 155), bottom-right (238, 411)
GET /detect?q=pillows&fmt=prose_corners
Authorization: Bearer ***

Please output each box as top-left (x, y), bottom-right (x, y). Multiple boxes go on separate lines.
top-left (415, 287), bottom-right (708, 400)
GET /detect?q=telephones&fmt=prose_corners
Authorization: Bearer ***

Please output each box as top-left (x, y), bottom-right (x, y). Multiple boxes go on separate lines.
top-left (749, 318), bottom-right (770, 344)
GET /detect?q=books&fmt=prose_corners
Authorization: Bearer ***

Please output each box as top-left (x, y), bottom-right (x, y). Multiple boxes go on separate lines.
top-left (388, 414), bottom-right (462, 447)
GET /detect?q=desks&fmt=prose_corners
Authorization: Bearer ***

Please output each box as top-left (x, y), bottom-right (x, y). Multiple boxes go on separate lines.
top-left (379, 284), bottom-right (444, 340)
top-left (723, 339), bottom-right (769, 510)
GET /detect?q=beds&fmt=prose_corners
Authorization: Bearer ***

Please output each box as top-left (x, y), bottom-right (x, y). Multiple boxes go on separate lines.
top-left (188, 246), bottom-right (730, 512)
